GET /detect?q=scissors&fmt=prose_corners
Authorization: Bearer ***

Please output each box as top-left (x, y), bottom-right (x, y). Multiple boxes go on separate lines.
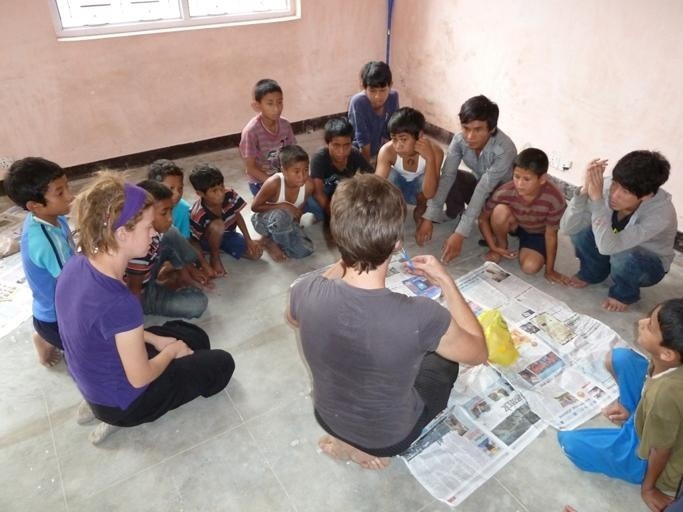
top-left (401, 247), bottom-right (414, 271)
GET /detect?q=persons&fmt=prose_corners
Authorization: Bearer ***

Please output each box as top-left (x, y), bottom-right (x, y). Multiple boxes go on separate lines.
top-left (5, 159), bottom-right (77, 368)
top-left (189, 161), bottom-right (265, 276)
top-left (415, 94), bottom-right (518, 265)
top-left (123, 159), bottom-right (217, 320)
top-left (238, 79), bottom-right (298, 197)
top-left (249, 145), bottom-right (316, 263)
top-left (559, 298), bottom-right (683, 512)
top-left (347, 60), bottom-right (400, 171)
top-left (302, 117), bottom-right (375, 223)
top-left (476, 148), bottom-right (569, 287)
top-left (372, 106), bottom-right (445, 223)
top-left (55, 167), bottom-right (235, 443)
top-left (558, 149), bottom-right (679, 312)
top-left (284, 173), bottom-right (488, 472)
